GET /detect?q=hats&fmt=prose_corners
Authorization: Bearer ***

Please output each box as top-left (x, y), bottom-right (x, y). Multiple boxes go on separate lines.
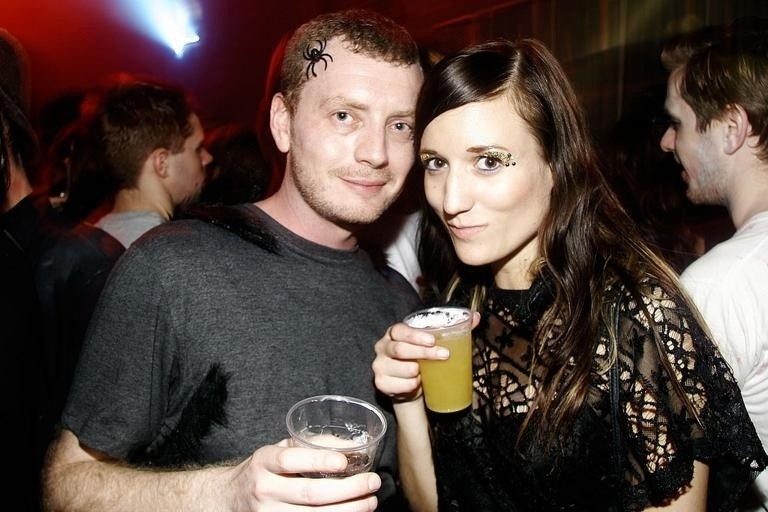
top-left (1, 34), bottom-right (41, 139)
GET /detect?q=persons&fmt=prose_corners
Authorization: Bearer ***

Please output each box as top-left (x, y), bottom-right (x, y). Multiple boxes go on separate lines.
top-left (0, 31), bottom-right (258, 511)
top-left (369, 36), bottom-right (768, 512)
top-left (651, 18), bottom-right (767, 512)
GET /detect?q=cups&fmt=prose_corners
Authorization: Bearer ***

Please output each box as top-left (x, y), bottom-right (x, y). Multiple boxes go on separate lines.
top-left (284, 392), bottom-right (389, 477)
top-left (404, 306), bottom-right (475, 412)
